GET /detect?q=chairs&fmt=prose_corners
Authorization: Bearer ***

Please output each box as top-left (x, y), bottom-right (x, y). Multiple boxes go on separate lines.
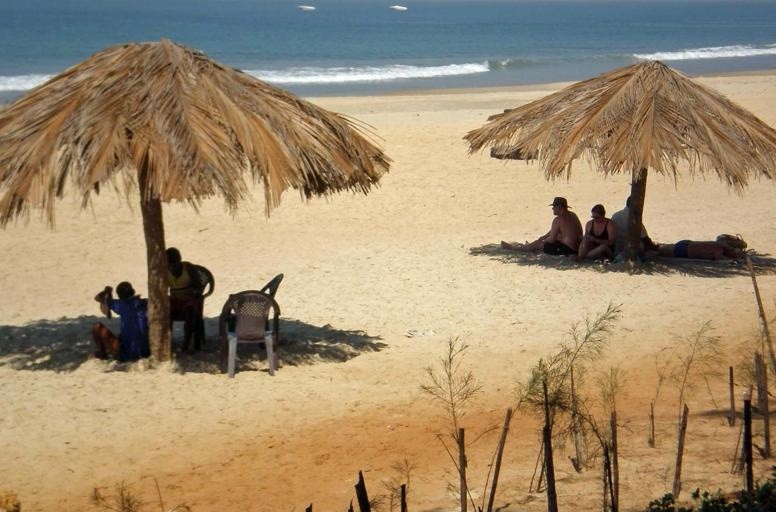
top-left (168, 264), bottom-right (284, 379)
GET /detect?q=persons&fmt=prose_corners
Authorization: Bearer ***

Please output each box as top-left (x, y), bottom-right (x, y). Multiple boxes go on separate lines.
top-left (610, 195), bottom-right (660, 257)
top-left (167, 246), bottom-right (208, 353)
top-left (88, 281), bottom-right (151, 362)
top-left (569, 202), bottom-right (617, 262)
top-left (500, 196), bottom-right (583, 255)
top-left (655, 234), bottom-right (750, 260)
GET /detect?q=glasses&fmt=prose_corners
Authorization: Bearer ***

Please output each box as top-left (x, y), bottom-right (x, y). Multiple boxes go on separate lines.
top-left (591, 216), bottom-right (602, 219)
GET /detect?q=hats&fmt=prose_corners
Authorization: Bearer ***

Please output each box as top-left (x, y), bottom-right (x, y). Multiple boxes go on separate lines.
top-left (548, 197), bottom-right (572, 208)
